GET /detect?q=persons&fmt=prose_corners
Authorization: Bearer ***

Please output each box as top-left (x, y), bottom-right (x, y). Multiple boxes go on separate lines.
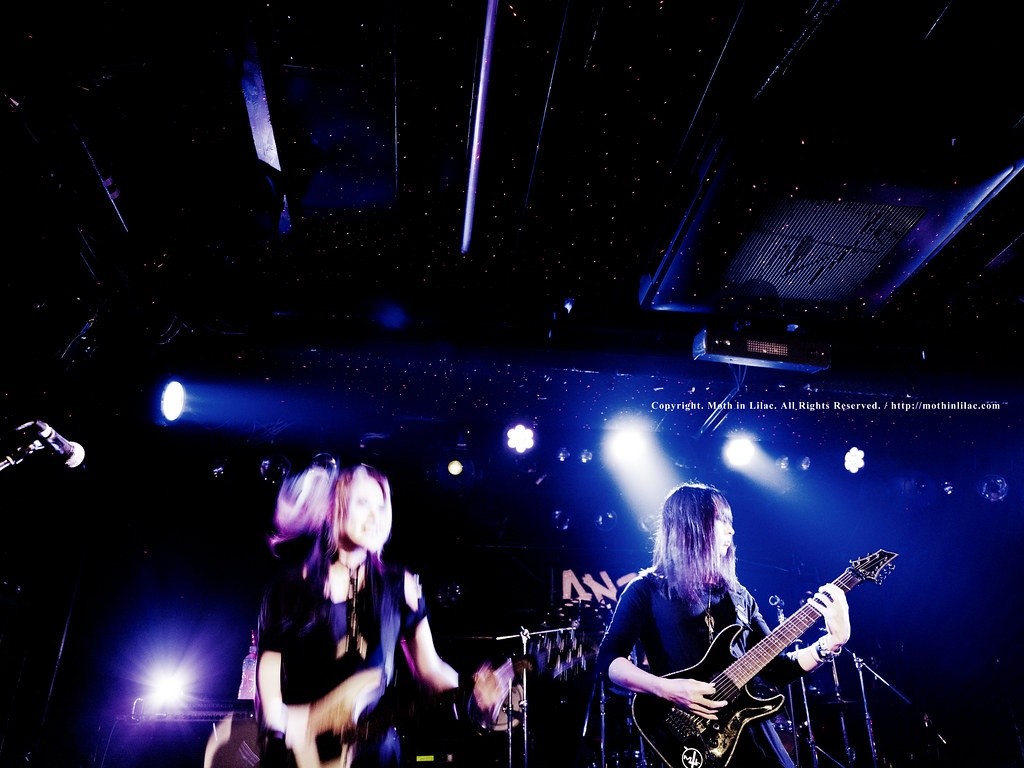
top-left (598, 483), bottom-right (851, 768)
top-left (256, 461), bottom-right (514, 768)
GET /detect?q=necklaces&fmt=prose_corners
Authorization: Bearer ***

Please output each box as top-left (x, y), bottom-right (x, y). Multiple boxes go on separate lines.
top-left (689, 583), bottom-right (715, 644)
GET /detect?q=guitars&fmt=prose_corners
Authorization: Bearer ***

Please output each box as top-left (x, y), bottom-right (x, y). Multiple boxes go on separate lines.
top-left (204, 663), bottom-right (505, 768)
top-left (632, 548), bottom-right (901, 768)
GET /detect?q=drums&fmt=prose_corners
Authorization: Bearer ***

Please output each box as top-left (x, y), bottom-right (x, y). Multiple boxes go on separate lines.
top-left (526, 601), bottom-right (616, 691)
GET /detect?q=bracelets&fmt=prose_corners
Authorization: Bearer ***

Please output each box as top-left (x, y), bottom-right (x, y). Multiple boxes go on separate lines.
top-left (811, 636), bottom-right (842, 664)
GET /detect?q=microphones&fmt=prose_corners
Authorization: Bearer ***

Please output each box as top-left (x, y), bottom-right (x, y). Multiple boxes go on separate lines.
top-left (768, 594), bottom-right (785, 609)
top-left (570, 619), bottom-right (580, 631)
top-left (33, 419), bottom-right (86, 471)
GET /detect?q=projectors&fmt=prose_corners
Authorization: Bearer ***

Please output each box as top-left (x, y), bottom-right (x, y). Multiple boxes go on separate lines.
top-left (691, 328), bottom-right (835, 374)
